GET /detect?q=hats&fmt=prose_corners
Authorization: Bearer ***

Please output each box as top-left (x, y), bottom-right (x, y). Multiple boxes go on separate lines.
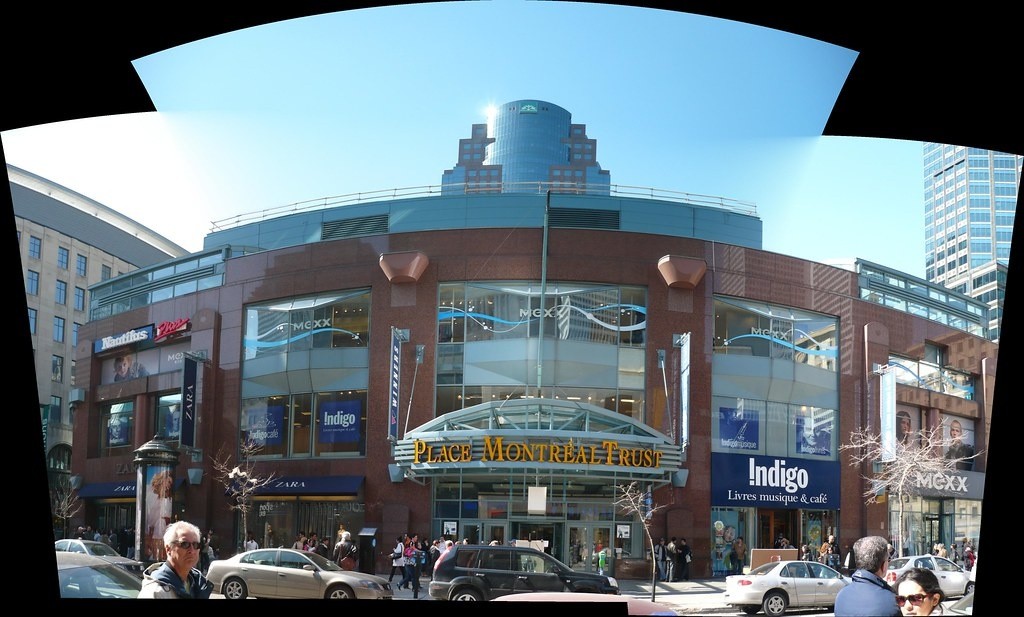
top-left (78, 527), bottom-right (83, 529)
top-left (965, 547), bottom-right (972, 552)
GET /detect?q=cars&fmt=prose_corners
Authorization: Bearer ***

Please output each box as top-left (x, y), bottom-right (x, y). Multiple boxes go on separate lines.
top-left (56, 551), bottom-right (144, 599)
top-left (943, 591), bottom-right (975, 616)
top-left (883, 554), bottom-right (975, 598)
top-left (54, 539), bottom-right (145, 578)
top-left (206, 546), bottom-right (395, 599)
top-left (725, 560), bottom-right (854, 617)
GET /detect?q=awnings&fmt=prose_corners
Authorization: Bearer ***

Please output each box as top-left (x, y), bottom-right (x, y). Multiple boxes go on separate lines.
top-left (76, 481), bottom-right (137, 498)
top-left (224, 475), bottom-right (365, 494)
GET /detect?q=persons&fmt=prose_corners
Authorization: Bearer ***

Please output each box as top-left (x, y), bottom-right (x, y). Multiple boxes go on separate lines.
top-left (138, 521), bottom-right (215, 600)
top-left (834, 536), bottom-right (901, 617)
top-left (942, 419), bottom-right (975, 472)
top-left (388, 533), bottom-right (517, 591)
top-left (112, 355), bottom-right (151, 382)
top-left (654, 536), bottom-right (693, 583)
top-left (894, 567), bottom-right (968, 617)
top-left (774, 533), bottom-right (977, 572)
top-left (897, 409), bottom-right (914, 453)
top-left (593, 539), bottom-right (603, 574)
top-left (72, 526), bottom-right (135, 560)
top-left (200, 526), bottom-right (359, 574)
top-left (335, 523), bottom-right (345, 545)
top-left (731, 536), bottom-right (749, 575)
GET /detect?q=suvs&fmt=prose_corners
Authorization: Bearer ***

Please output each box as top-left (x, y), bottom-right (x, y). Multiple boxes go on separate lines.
top-left (428, 546), bottom-right (622, 603)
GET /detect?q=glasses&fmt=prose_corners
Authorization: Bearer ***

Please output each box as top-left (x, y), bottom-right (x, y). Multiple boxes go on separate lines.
top-left (895, 594), bottom-right (932, 606)
top-left (181, 542), bottom-right (198, 549)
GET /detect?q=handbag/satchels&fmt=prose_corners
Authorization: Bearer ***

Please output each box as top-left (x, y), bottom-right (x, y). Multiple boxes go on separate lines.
top-left (390, 544), bottom-right (402, 559)
top-left (685, 554), bottom-right (691, 563)
top-left (339, 557), bottom-right (356, 571)
top-left (404, 546), bottom-right (416, 557)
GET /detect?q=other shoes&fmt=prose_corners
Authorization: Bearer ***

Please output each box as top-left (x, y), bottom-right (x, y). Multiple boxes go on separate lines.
top-left (397, 584), bottom-right (401, 590)
top-left (404, 587), bottom-right (409, 589)
